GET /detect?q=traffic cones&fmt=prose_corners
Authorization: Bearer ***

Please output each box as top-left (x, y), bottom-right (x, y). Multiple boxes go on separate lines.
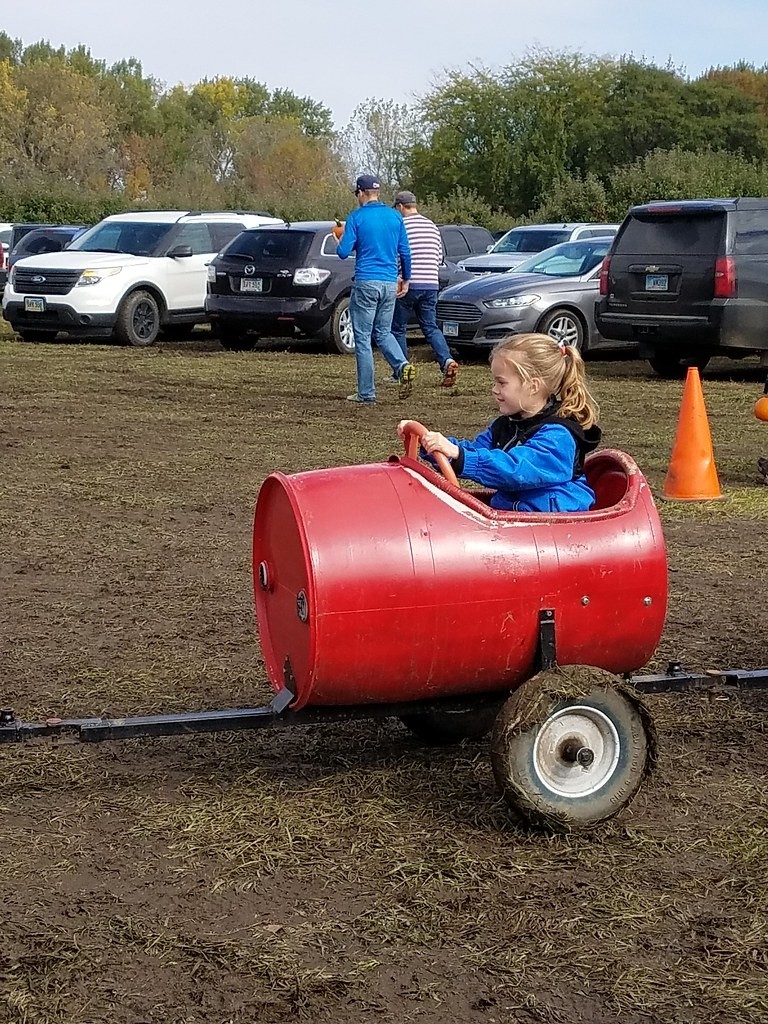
top-left (655, 368), bottom-right (726, 501)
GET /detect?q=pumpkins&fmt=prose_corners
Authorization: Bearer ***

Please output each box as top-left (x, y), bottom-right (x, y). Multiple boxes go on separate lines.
top-left (755, 397), bottom-right (768, 421)
top-left (332, 218), bottom-right (345, 238)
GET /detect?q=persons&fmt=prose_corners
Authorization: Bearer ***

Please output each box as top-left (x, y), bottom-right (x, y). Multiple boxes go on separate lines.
top-left (333, 175), bottom-right (414, 401)
top-left (396, 331), bottom-right (603, 513)
top-left (383, 191), bottom-right (459, 387)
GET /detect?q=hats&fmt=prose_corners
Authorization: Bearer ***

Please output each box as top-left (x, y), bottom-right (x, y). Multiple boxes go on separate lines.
top-left (391, 191), bottom-right (417, 208)
top-left (352, 174), bottom-right (381, 193)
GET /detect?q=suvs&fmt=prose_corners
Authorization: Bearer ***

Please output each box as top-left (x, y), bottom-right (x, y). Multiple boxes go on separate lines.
top-left (456, 221), bottom-right (622, 276)
top-left (435, 222), bottom-right (495, 261)
top-left (2, 208), bottom-right (284, 346)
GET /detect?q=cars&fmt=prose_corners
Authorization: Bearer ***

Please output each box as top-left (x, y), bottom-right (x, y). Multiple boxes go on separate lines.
top-left (6, 227), bottom-right (88, 275)
top-left (203, 221), bottom-right (476, 355)
top-left (593, 196), bottom-right (768, 380)
top-left (434, 235), bottom-right (641, 364)
top-left (0, 222), bottom-right (18, 269)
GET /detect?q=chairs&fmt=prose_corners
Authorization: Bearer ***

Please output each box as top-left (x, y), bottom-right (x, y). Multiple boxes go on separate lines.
top-left (119, 233), bottom-right (136, 251)
top-left (461, 448), bottom-right (637, 516)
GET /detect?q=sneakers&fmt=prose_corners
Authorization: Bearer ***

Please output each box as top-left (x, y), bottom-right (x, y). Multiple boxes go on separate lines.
top-left (347, 393), bottom-right (365, 403)
top-left (399, 365), bottom-right (415, 399)
top-left (442, 359), bottom-right (459, 387)
top-left (383, 376), bottom-right (398, 383)
